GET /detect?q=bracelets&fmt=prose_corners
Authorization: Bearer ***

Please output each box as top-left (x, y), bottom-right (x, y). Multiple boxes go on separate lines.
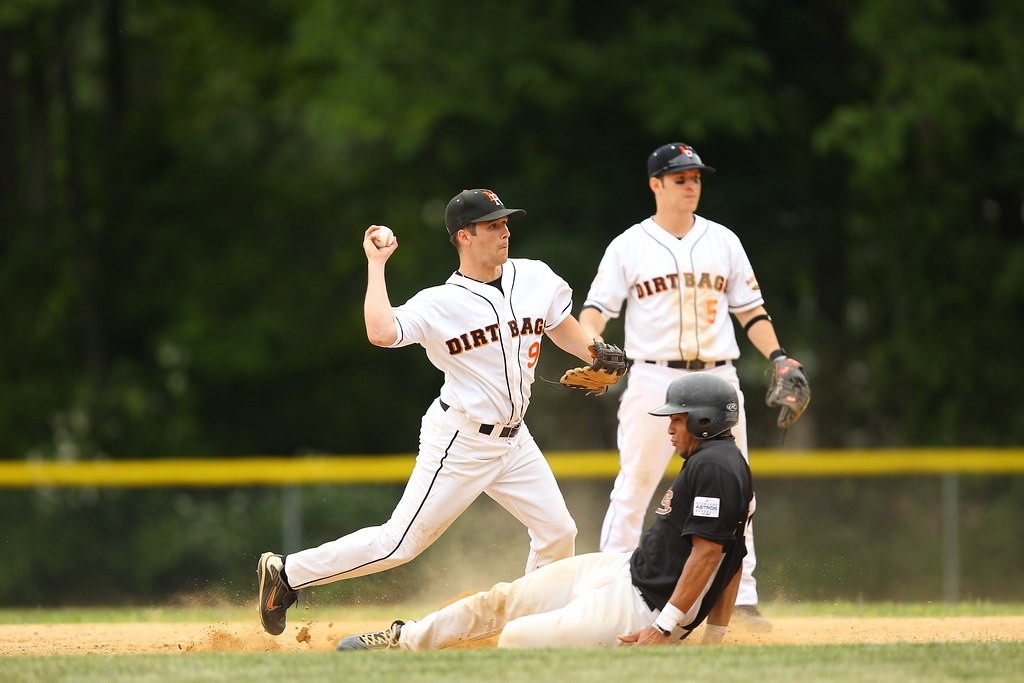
top-left (703, 624), bottom-right (727, 646)
top-left (652, 602), bottom-right (685, 637)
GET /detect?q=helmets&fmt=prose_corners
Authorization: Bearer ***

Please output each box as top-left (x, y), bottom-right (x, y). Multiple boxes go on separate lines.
top-left (648, 372), bottom-right (739, 439)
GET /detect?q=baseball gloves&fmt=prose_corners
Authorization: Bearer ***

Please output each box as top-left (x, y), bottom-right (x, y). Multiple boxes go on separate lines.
top-left (766, 358), bottom-right (811, 427)
top-left (560, 343), bottom-right (629, 391)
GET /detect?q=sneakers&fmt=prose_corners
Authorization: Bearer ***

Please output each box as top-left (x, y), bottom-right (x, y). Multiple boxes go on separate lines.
top-left (336, 620), bottom-right (405, 651)
top-left (256, 552), bottom-right (302, 635)
top-left (733, 605), bottom-right (773, 633)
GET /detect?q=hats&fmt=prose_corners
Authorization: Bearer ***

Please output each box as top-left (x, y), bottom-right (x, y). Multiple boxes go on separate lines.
top-left (445, 189), bottom-right (526, 236)
top-left (647, 142), bottom-right (716, 179)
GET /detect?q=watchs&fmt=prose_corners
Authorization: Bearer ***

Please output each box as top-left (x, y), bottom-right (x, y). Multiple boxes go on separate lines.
top-left (653, 621), bottom-right (671, 637)
top-left (769, 348), bottom-right (786, 361)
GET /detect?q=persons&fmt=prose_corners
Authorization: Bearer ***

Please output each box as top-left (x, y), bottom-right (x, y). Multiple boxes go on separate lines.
top-left (256, 189), bottom-right (629, 636)
top-left (336, 373), bottom-right (755, 646)
top-left (578, 142), bottom-right (810, 625)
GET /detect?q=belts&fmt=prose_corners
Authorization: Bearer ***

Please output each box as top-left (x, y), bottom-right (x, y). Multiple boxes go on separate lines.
top-left (440, 398), bottom-right (521, 439)
top-left (639, 588), bottom-right (692, 640)
top-left (629, 359), bottom-right (725, 369)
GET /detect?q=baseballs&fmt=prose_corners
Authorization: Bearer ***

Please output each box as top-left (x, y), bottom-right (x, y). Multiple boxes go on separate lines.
top-left (371, 226), bottom-right (394, 247)
